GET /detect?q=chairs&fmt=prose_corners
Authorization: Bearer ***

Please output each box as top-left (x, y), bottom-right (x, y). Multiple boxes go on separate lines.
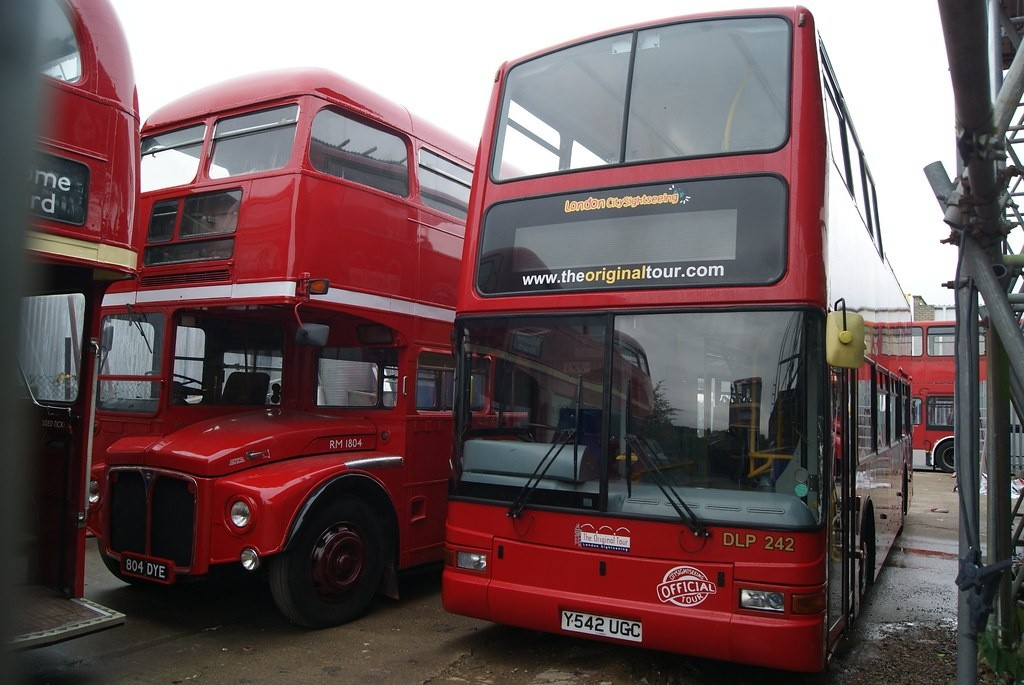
top-left (550, 406), bottom-right (602, 466)
top-left (222, 372), bottom-right (270, 405)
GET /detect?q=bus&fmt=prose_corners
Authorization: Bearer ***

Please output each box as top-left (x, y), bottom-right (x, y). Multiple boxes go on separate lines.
top-left (912, 321), bottom-right (987, 473)
top-left (545, 321), bottom-right (655, 436)
top-left (93, 66), bottom-right (539, 633)
top-left (13, 0), bottom-right (140, 651)
top-left (440, 6), bottom-right (912, 672)
top-left (831, 320), bottom-right (912, 471)
